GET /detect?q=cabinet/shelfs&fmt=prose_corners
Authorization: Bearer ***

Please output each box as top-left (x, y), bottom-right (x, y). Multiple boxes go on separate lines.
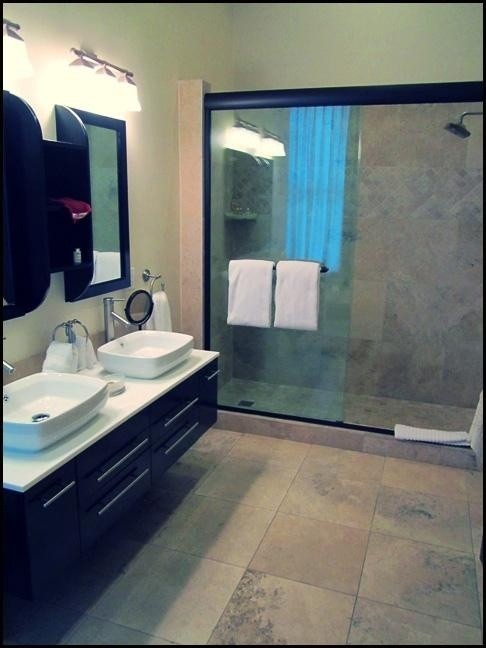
top-left (201, 356), bottom-right (218, 435)
top-left (78, 403), bottom-right (151, 557)
top-left (22, 455), bottom-right (78, 606)
top-left (151, 369), bottom-right (201, 488)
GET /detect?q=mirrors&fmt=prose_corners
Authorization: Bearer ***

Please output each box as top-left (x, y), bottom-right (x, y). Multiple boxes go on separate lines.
top-left (54, 103), bottom-right (132, 305)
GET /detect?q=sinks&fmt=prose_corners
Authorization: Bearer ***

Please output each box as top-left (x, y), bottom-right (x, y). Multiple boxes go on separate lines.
top-left (2, 371), bottom-right (107, 452)
top-left (96, 328), bottom-right (195, 377)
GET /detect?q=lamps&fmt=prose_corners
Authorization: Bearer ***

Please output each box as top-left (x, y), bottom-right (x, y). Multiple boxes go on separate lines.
top-left (220, 117), bottom-right (288, 161)
top-left (54, 47), bottom-right (143, 115)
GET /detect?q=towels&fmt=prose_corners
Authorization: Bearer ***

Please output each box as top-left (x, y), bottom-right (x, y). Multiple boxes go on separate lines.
top-left (226, 258), bottom-right (322, 332)
top-left (41, 335), bottom-right (98, 374)
top-left (145, 290), bottom-right (172, 332)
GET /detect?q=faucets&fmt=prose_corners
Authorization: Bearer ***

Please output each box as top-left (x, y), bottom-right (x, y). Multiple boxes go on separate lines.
top-left (1, 338), bottom-right (16, 375)
top-left (102, 297), bottom-right (130, 341)
